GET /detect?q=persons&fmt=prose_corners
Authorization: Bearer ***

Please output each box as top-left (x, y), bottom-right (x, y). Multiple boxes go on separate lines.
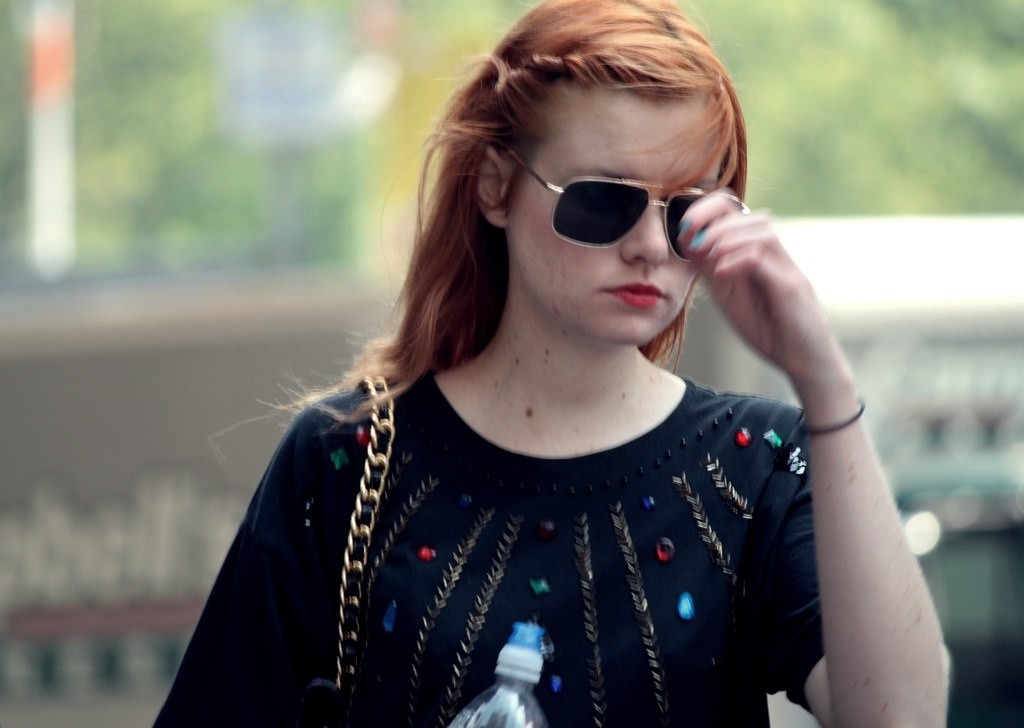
top-left (149, 0), bottom-right (951, 728)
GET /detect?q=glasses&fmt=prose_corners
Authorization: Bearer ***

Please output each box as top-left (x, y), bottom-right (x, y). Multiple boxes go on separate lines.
top-left (506, 148), bottom-right (751, 262)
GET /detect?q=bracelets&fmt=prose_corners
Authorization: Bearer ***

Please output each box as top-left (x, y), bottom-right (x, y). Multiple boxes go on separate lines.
top-left (797, 398), bottom-right (865, 436)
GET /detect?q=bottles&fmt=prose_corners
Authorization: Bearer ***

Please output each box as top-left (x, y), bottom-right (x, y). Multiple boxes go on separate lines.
top-left (446, 622), bottom-right (549, 727)
